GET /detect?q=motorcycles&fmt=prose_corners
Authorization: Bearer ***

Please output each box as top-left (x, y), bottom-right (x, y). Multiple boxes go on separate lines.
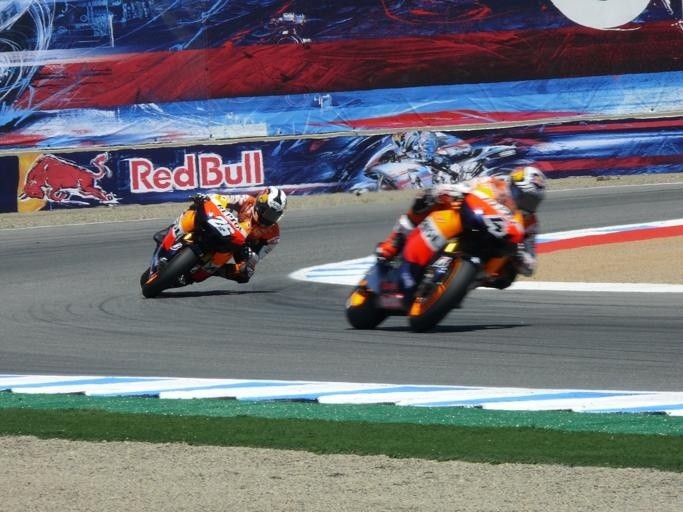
top-left (345, 189), bottom-right (526, 332)
top-left (141, 194), bottom-right (250, 298)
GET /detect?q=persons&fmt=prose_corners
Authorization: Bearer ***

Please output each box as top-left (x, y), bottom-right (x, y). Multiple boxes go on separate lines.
top-left (393, 129), bottom-right (472, 183)
top-left (152, 184), bottom-right (287, 276)
top-left (374, 166), bottom-right (545, 315)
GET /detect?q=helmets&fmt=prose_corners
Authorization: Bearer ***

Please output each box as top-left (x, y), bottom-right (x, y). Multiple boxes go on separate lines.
top-left (507, 165), bottom-right (548, 216)
top-left (253, 185), bottom-right (289, 226)
